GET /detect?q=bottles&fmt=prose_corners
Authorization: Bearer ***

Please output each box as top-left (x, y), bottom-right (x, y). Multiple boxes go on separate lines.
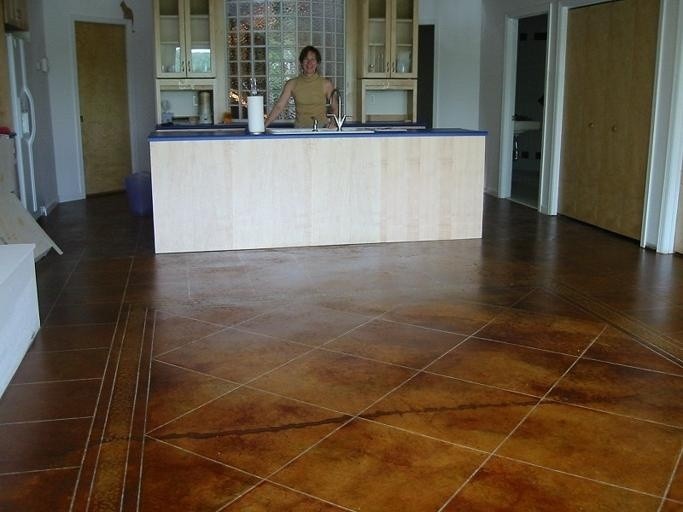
top-left (368, 46), bottom-right (383, 72)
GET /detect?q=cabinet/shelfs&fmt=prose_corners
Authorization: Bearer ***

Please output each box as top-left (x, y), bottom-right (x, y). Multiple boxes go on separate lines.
top-left (358, 0), bottom-right (418, 123)
top-left (152, 0), bottom-right (226, 125)
top-left (558, 0), bottom-right (661, 241)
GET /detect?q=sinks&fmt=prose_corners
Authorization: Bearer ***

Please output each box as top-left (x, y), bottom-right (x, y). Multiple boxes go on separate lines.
top-left (265, 126), bottom-right (375, 136)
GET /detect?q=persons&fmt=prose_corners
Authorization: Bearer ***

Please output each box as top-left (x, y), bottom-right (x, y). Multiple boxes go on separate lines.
top-left (264, 46), bottom-right (339, 129)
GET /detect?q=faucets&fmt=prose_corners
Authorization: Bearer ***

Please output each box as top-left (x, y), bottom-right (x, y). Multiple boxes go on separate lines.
top-left (325, 88), bottom-right (354, 132)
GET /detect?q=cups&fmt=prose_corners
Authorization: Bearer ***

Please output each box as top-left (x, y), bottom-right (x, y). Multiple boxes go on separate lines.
top-left (399, 63), bottom-right (407, 72)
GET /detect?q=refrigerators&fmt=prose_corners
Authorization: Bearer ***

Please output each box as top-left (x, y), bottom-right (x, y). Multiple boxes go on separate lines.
top-left (5, 32), bottom-right (60, 221)
